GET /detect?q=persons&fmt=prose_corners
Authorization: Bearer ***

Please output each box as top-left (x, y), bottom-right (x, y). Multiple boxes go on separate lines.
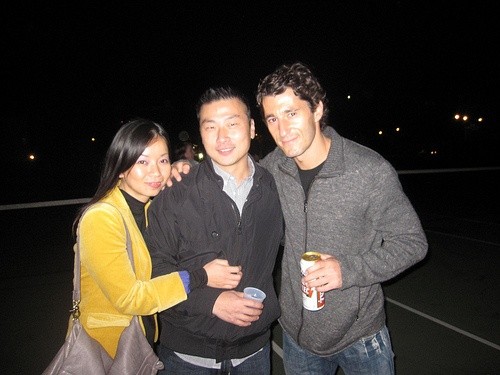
top-left (41, 119), bottom-right (243, 372)
top-left (144, 88), bottom-right (284, 372)
top-left (161, 63), bottom-right (428, 372)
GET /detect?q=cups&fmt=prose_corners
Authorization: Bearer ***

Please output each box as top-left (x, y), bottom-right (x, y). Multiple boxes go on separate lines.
top-left (243, 287), bottom-right (266, 303)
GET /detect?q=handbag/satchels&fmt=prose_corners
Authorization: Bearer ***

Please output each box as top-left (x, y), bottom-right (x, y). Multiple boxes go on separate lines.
top-left (40, 317), bottom-right (160, 375)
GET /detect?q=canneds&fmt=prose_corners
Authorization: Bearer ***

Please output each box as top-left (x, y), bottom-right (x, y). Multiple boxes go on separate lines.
top-left (300, 251), bottom-right (325, 311)
